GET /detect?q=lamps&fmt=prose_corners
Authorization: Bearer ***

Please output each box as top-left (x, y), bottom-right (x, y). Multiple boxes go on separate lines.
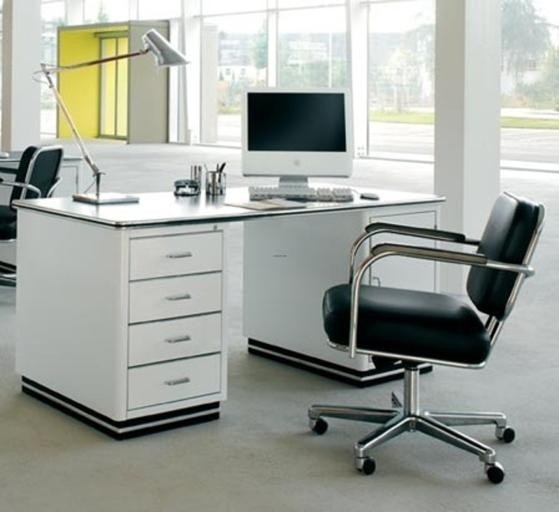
top-left (32, 29), bottom-right (188, 206)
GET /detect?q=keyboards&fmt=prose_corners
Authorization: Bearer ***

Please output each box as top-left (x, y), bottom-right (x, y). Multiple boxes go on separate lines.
top-left (248, 186), bottom-right (353, 202)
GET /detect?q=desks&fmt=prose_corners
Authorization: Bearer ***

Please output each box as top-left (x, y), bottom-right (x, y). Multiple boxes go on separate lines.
top-left (12, 178), bottom-right (447, 441)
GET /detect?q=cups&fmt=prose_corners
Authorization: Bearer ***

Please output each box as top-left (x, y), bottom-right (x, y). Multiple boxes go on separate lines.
top-left (207, 171), bottom-right (227, 195)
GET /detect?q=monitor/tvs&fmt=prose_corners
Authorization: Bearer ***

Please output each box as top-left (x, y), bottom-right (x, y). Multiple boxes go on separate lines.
top-left (242, 88), bottom-right (354, 188)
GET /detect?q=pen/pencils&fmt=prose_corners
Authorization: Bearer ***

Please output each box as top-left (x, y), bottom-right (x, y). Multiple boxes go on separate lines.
top-left (204, 163), bottom-right (225, 195)
top-left (191, 166), bottom-right (201, 183)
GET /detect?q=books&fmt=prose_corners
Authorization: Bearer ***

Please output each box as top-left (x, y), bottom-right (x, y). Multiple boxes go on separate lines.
top-left (225, 196), bottom-right (306, 211)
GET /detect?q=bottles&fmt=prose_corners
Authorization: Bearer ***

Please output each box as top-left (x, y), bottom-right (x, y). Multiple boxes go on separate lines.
top-left (190, 165), bottom-right (202, 191)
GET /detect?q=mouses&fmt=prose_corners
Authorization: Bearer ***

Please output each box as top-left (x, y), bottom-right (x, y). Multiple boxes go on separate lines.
top-left (360, 193), bottom-right (379, 200)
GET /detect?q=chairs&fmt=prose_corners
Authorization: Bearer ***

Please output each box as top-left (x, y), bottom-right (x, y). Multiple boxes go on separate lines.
top-left (308, 192), bottom-right (546, 483)
top-left (0, 145), bottom-right (64, 286)
